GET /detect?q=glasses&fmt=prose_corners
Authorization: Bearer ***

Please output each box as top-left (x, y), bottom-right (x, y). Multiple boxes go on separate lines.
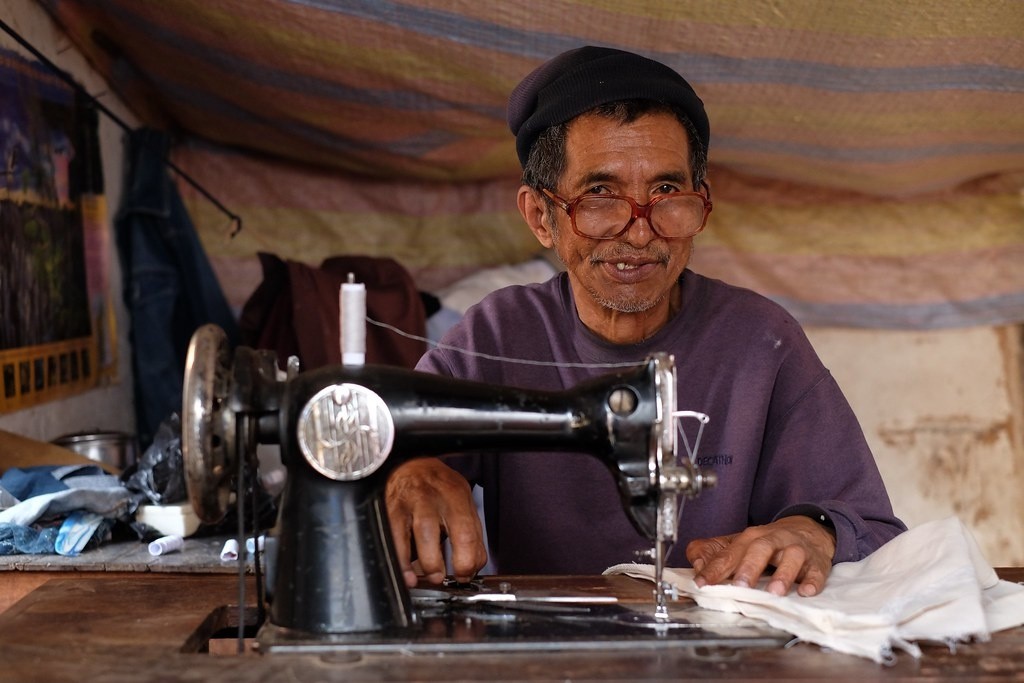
top-left (525, 171), bottom-right (712, 241)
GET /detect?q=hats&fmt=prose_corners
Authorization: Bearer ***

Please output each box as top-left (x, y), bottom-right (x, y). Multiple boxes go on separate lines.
top-left (505, 45), bottom-right (711, 168)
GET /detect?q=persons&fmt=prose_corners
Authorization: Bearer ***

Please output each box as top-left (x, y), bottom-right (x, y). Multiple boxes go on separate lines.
top-left (386, 45), bottom-right (909, 599)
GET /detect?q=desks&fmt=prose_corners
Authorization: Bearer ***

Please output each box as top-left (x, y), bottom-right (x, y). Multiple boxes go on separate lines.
top-left (0, 566), bottom-right (1024, 683)
